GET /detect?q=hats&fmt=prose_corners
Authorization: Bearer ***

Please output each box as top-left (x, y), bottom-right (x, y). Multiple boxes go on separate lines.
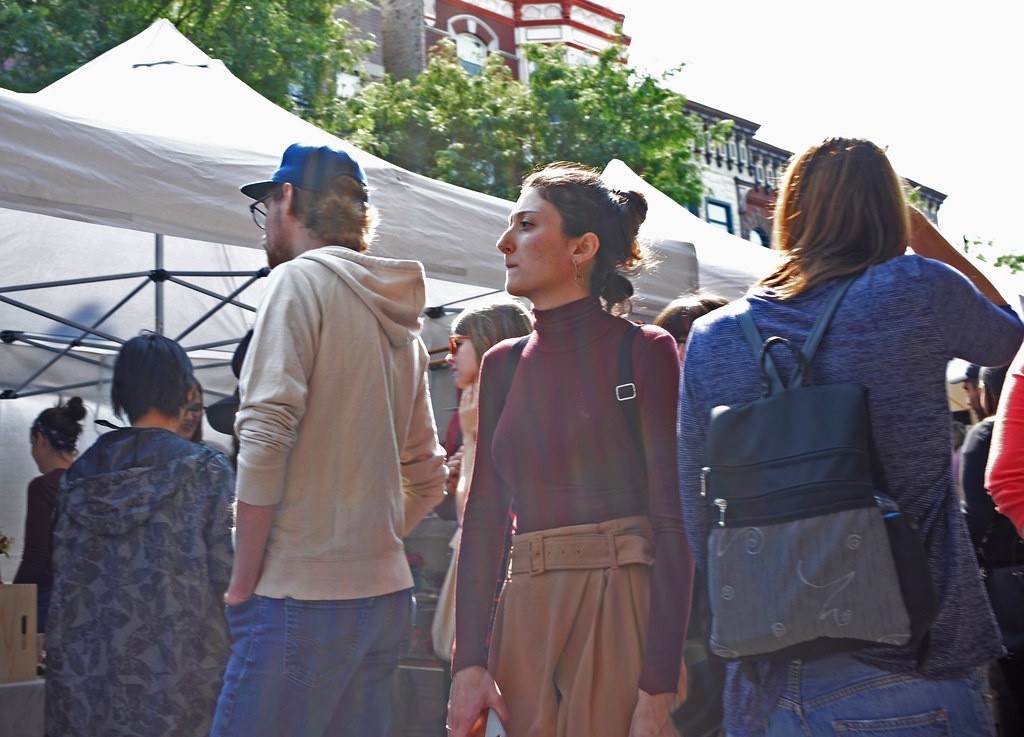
top-left (239, 142), bottom-right (368, 205)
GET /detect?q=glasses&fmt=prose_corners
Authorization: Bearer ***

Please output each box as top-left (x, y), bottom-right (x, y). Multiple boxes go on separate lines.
top-left (185, 404), bottom-right (207, 417)
top-left (449, 333), bottom-right (470, 354)
top-left (250, 193), bottom-right (274, 230)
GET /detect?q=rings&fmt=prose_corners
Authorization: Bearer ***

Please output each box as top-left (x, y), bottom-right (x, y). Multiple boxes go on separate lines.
top-left (445, 723), bottom-right (453, 731)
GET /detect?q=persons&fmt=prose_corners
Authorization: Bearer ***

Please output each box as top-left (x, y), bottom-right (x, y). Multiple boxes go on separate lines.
top-left (204, 142), bottom-right (452, 737)
top-left (12, 280), bottom-right (1024, 735)
top-left (441, 159), bottom-right (699, 737)
top-left (678, 135), bottom-right (1024, 737)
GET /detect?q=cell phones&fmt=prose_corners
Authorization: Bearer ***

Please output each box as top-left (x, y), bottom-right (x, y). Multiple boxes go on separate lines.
top-left (465, 707), bottom-right (507, 737)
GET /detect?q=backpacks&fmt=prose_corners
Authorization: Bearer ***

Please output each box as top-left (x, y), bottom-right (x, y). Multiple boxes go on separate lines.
top-left (700, 268), bottom-right (942, 686)
